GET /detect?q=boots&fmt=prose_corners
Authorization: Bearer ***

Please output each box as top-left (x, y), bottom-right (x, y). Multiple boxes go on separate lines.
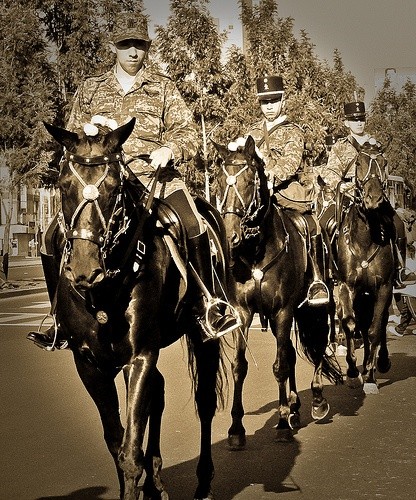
top-left (188, 225), bottom-right (236, 343)
top-left (392, 236), bottom-right (406, 289)
top-left (26, 245), bottom-right (67, 347)
top-left (306, 234), bottom-right (328, 307)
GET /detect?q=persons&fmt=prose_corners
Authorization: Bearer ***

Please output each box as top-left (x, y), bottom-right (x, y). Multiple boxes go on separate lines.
top-left (29, 238), bottom-right (38, 257)
top-left (394, 203), bottom-right (400, 210)
top-left (389, 244), bottom-right (416, 337)
top-left (26, 13), bottom-right (235, 346)
top-left (244, 75), bottom-right (327, 301)
top-left (322, 101), bottom-right (416, 289)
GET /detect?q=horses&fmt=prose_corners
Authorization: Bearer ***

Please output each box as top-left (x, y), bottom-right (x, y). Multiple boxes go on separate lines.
top-left (334, 133), bottom-right (401, 396)
top-left (42, 120), bottom-right (226, 500)
top-left (312, 170), bottom-right (345, 278)
top-left (208, 136), bottom-right (331, 450)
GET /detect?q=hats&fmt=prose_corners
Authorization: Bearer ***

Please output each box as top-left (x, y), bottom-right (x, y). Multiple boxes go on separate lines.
top-left (112, 12), bottom-right (150, 44)
top-left (256, 76), bottom-right (285, 101)
top-left (344, 91), bottom-right (366, 120)
